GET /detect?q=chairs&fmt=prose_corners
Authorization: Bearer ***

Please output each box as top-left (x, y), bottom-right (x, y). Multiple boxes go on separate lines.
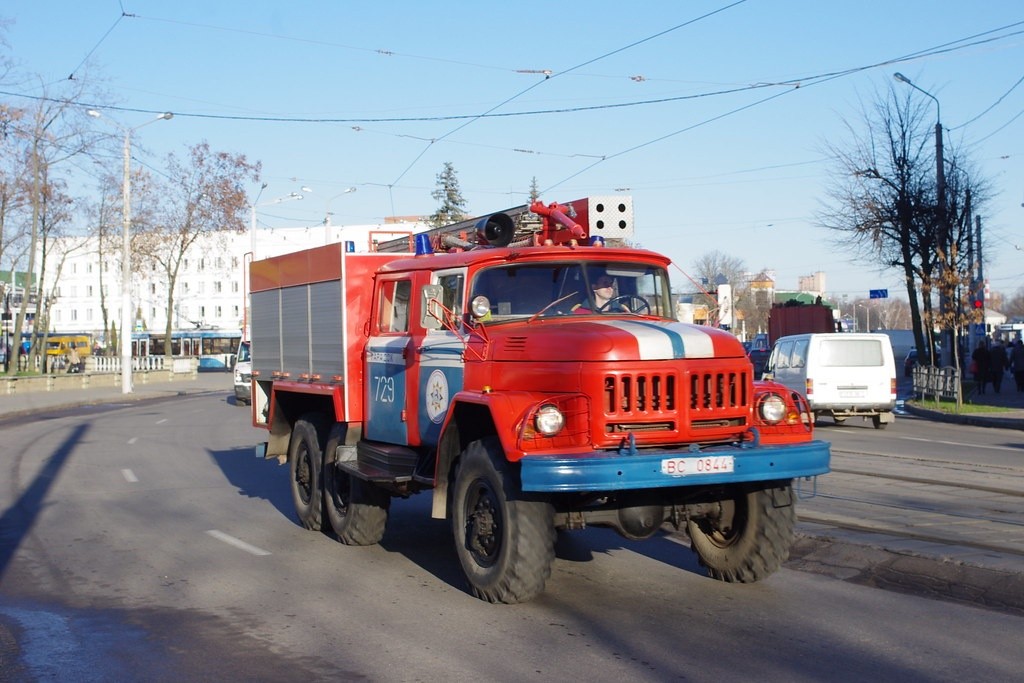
top-left (552, 278), bottom-right (588, 315)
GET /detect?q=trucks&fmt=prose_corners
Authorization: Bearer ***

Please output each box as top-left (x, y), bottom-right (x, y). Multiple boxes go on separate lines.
top-left (768, 306), bottom-right (838, 342)
top-left (250, 197), bottom-right (835, 604)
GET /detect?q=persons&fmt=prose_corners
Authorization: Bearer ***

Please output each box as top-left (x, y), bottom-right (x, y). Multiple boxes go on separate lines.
top-left (573, 271), bottom-right (630, 314)
top-left (93, 342), bottom-right (100, 351)
top-left (66, 345), bottom-right (80, 374)
top-left (1006, 340), bottom-right (1024, 394)
top-left (972, 341), bottom-right (990, 395)
top-left (990, 342), bottom-right (1008, 393)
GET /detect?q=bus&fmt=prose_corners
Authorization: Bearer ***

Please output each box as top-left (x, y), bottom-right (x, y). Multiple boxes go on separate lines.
top-left (132, 333), bottom-right (240, 371)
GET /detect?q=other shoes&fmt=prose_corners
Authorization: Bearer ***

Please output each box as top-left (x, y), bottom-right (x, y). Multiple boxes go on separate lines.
top-left (1017, 387), bottom-right (1019, 392)
top-left (978, 391), bottom-right (981, 395)
top-left (983, 390), bottom-right (984, 393)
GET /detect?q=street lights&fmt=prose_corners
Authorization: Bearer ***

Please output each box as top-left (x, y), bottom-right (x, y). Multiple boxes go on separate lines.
top-left (893, 71), bottom-right (957, 366)
top-left (301, 186), bottom-right (357, 243)
top-left (87, 109), bottom-right (174, 392)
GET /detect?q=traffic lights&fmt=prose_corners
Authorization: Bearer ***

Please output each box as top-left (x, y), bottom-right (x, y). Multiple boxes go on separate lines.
top-left (974, 298), bottom-right (984, 310)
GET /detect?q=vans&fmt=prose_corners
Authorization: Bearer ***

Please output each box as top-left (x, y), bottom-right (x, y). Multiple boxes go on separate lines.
top-left (234, 337), bottom-right (254, 405)
top-left (38, 335), bottom-right (92, 358)
top-left (771, 330), bottom-right (897, 431)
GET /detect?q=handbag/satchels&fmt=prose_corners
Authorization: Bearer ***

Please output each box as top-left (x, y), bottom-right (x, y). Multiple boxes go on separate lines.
top-left (970, 360), bottom-right (977, 374)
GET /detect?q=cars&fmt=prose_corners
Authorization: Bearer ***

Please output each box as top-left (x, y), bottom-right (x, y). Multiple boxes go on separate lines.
top-left (906, 348), bottom-right (943, 377)
top-left (743, 334), bottom-right (775, 378)
